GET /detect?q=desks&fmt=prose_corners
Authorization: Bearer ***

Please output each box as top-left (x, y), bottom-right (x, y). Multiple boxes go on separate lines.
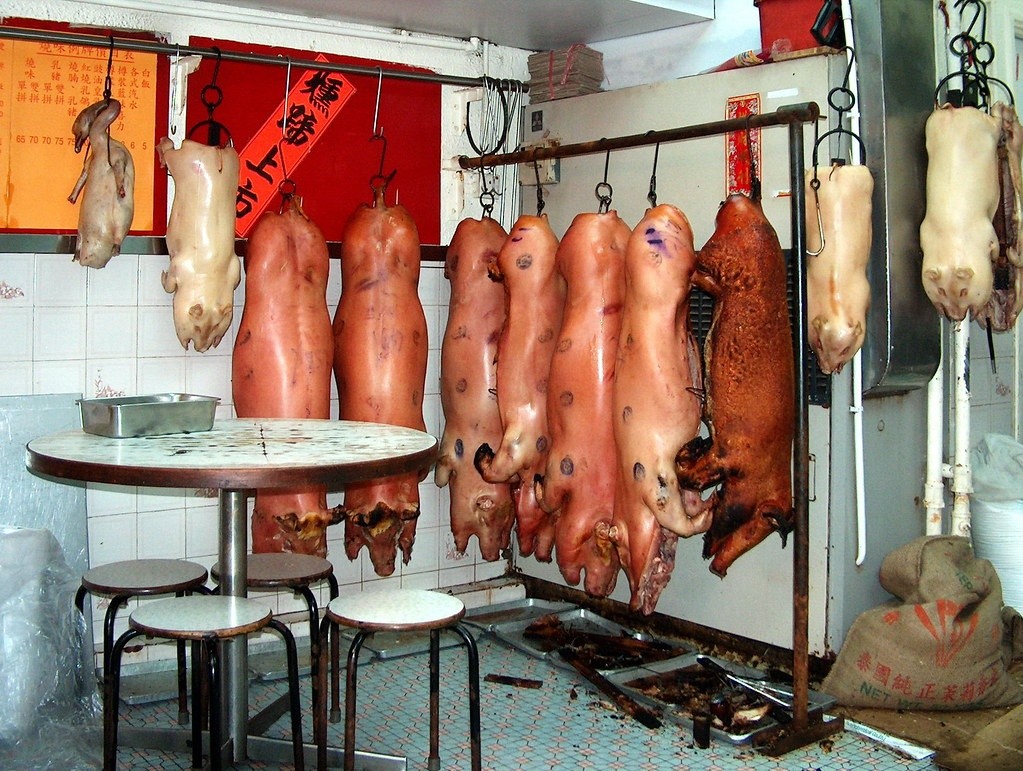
top-left (23, 416), bottom-right (439, 771)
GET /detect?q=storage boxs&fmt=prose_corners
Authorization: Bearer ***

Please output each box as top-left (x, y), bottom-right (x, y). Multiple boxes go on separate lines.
top-left (753, 0), bottom-right (841, 64)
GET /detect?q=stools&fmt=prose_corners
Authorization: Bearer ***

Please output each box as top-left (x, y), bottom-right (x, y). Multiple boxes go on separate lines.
top-left (74, 552), bottom-right (481, 771)
